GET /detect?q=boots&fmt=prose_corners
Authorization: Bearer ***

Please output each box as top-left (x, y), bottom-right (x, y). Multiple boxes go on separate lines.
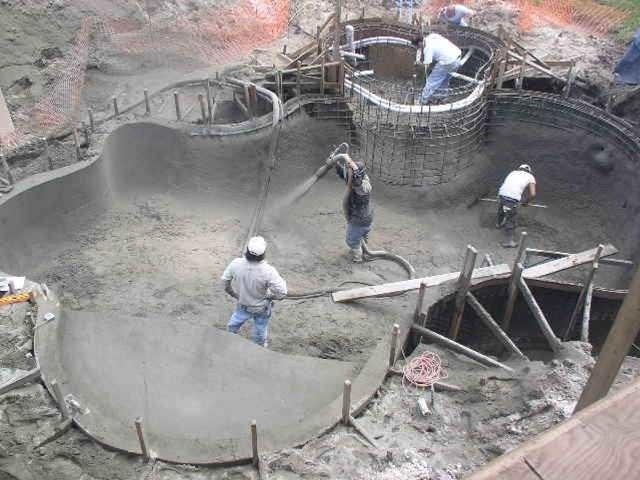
top-left (495, 218), bottom-right (519, 248)
top-left (350, 246), bottom-right (362, 262)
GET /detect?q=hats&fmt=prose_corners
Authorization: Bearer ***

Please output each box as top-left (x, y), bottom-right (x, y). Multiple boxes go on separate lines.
top-left (247, 235), bottom-right (268, 257)
top-left (519, 164), bottom-right (532, 173)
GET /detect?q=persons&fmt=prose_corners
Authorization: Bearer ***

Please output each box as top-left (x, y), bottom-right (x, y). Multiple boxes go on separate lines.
top-left (437, 5), bottom-right (477, 26)
top-left (222, 236), bottom-right (287, 348)
top-left (412, 34), bottom-right (461, 105)
top-left (335, 160), bottom-right (374, 264)
top-left (496, 164), bottom-right (537, 248)
